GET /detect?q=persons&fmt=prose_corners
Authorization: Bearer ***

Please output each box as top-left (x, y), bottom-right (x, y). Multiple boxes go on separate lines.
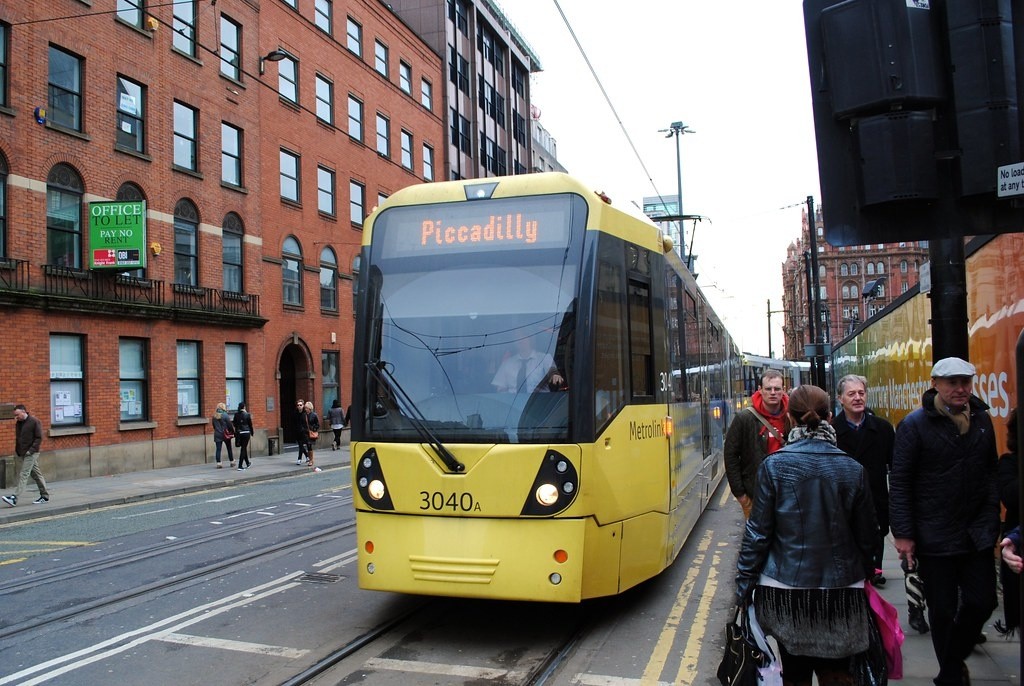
top-left (490, 322), bottom-right (564, 393)
top-left (344, 404), bottom-right (352, 439)
top-left (232, 402), bottom-right (254, 471)
top-left (305, 401), bottom-right (320, 466)
top-left (994, 407), bottom-right (1024, 640)
top-left (889, 357), bottom-right (1001, 686)
top-left (999, 523), bottom-right (1024, 574)
top-left (715, 385), bottom-right (905, 686)
top-left (830, 374), bottom-right (896, 585)
top-left (724, 369), bottom-right (791, 520)
top-left (294, 399), bottom-right (310, 465)
top-left (2, 404), bottom-right (49, 507)
top-left (212, 402), bottom-right (237, 469)
top-left (327, 399), bottom-right (345, 451)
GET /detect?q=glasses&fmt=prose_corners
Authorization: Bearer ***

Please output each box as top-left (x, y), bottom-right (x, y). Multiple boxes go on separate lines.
top-left (297, 404), bottom-right (302, 406)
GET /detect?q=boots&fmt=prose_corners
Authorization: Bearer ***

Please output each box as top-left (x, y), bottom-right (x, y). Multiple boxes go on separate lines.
top-left (308, 451), bottom-right (314, 465)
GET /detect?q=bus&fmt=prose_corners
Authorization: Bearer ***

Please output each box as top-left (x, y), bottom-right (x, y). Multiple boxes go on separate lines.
top-left (346, 170), bottom-right (811, 605)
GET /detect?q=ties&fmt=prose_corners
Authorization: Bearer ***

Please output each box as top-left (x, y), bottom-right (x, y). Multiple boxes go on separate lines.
top-left (517, 359), bottom-right (529, 393)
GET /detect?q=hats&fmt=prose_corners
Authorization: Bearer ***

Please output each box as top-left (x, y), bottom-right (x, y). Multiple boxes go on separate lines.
top-left (931, 357), bottom-right (975, 377)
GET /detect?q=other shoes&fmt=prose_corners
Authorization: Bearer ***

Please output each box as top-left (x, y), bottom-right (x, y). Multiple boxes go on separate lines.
top-left (332, 441), bottom-right (336, 451)
top-left (237, 467), bottom-right (245, 471)
top-left (247, 462), bottom-right (252, 468)
top-left (306, 457), bottom-right (309, 462)
top-left (230, 459), bottom-right (237, 467)
top-left (337, 446), bottom-right (340, 450)
top-left (297, 460), bottom-right (301, 465)
top-left (216, 461), bottom-right (223, 469)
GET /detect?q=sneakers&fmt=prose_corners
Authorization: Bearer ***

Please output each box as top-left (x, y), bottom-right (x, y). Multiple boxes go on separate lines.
top-left (2, 495), bottom-right (18, 507)
top-left (32, 497), bottom-right (50, 504)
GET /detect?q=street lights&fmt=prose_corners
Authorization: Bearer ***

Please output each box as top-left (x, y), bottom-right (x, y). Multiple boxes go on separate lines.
top-left (659, 122), bottom-right (694, 260)
top-left (766, 299), bottom-right (792, 358)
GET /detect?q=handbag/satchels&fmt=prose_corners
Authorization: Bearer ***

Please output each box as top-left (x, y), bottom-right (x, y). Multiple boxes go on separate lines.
top-left (309, 431), bottom-right (319, 439)
top-left (865, 585), bottom-right (905, 686)
top-left (223, 429), bottom-right (234, 440)
top-left (716, 606), bottom-right (780, 686)
top-left (235, 432), bottom-right (241, 447)
